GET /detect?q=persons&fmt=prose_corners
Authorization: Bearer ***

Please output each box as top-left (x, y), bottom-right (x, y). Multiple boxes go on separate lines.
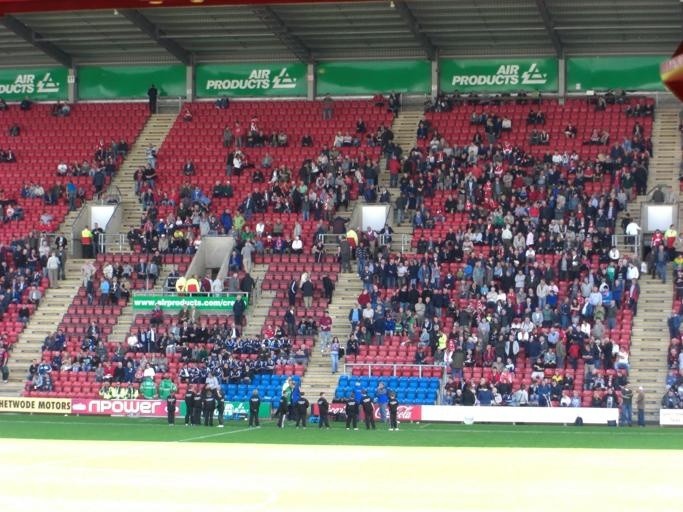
top-left (0, 82), bottom-right (683, 432)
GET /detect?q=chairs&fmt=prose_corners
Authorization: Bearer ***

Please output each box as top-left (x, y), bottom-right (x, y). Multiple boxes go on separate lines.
top-left (0, 95), bottom-right (656, 411)
